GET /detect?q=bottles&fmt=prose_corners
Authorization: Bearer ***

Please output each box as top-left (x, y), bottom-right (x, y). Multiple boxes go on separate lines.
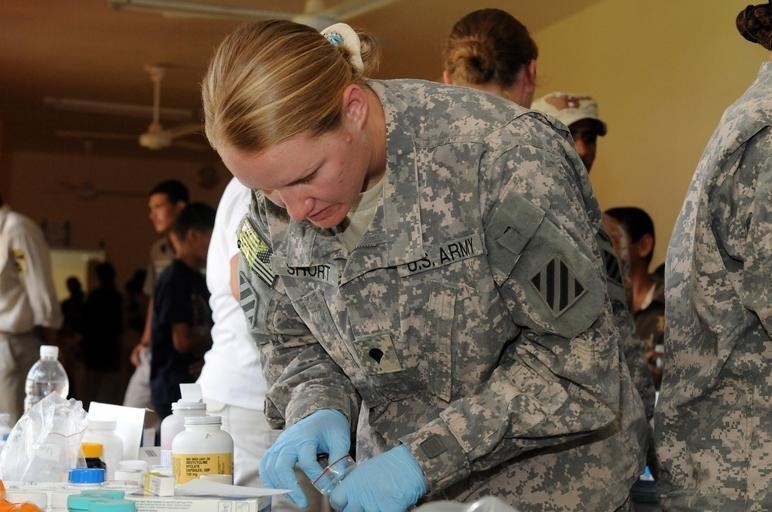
top-left (1, 342), bottom-right (236, 511)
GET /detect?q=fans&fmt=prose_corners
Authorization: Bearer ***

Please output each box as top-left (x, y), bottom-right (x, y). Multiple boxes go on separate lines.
top-left (54, 65), bottom-right (202, 152)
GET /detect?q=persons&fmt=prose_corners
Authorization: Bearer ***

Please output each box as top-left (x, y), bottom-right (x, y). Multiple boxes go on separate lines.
top-left (202, 17), bottom-right (654, 512)
top-left (602, 205), bottom-right (665, 441)
top-left (193, 174), bottom-right (323, 512)
top-left (652, 2), bottom-right (772, 511)
top-left (1, 180), bottom-right (213, 442)
top-left (441, 8), bottom-right (541, 108)
top-left (531, 92), bottom-right (607, 176)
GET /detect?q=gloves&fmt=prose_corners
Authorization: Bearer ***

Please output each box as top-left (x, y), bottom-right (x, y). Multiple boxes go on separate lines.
top-left (329, 444), bottom-right (428, 512)
top-left (258, 409), bottom-right (350, 509)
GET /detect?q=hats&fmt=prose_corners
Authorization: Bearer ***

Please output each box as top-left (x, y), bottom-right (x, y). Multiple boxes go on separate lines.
top-left (531, 93), bottom-right (606, 136)
